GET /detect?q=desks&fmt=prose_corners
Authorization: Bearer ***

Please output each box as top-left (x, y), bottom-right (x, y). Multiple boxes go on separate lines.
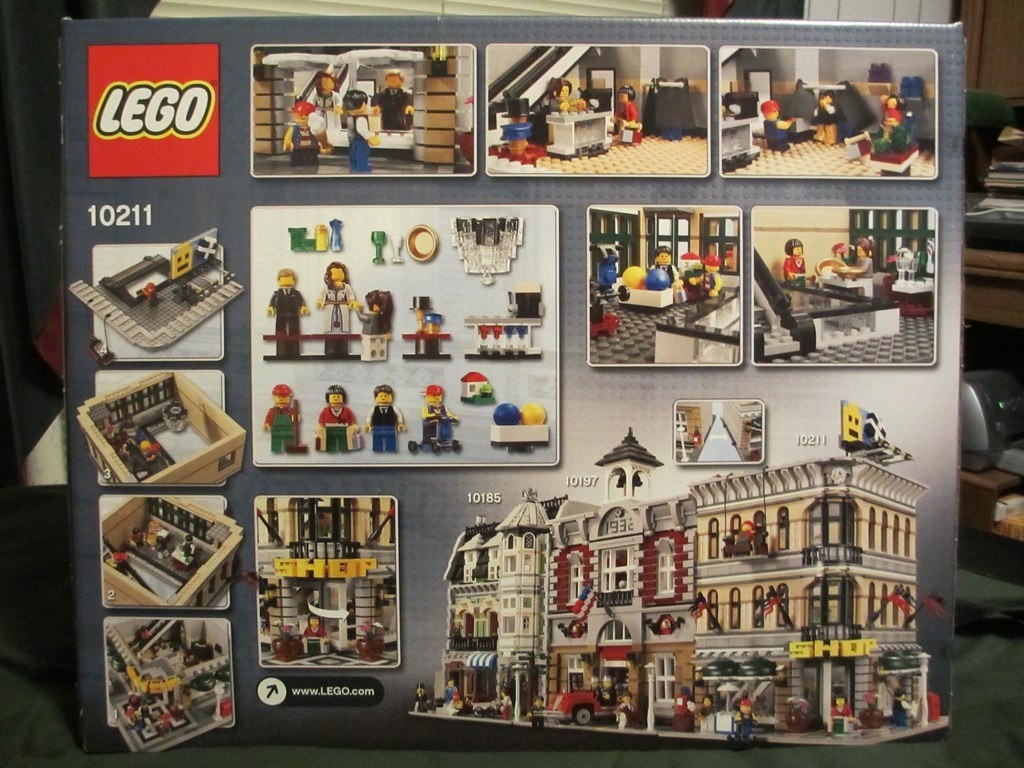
top-left (963, 247), bottom-right (1024, 332)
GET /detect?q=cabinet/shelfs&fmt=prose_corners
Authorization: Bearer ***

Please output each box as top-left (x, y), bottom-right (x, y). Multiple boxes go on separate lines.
top-left (956, 0), bottom-right (1024, 108)
